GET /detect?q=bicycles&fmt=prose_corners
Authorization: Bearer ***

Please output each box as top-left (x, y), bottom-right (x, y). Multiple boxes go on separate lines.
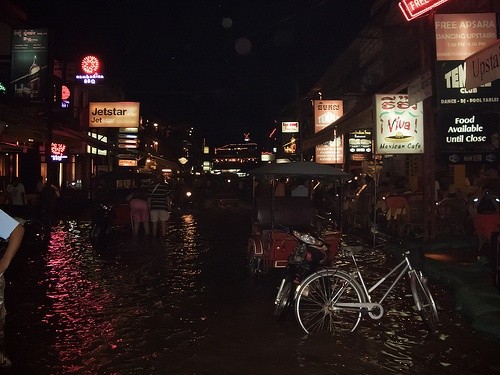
top-left (294, 239), bottom-right (437, 338)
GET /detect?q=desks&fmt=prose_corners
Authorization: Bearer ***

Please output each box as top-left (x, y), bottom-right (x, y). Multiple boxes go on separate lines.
top-left (472, 212), bottom-right (500, 251)
top-left (369, 190), bottom-right (500, 251)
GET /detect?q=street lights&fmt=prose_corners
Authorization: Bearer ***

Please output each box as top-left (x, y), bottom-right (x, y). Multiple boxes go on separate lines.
top-left (178, 149), bottom-right (189, 180)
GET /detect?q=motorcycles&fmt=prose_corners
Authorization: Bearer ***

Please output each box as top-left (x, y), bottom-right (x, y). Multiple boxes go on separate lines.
top-left (263, 224), bottom-right (357, 321)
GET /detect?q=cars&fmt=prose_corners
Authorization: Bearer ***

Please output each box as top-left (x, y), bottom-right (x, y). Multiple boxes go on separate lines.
top-left (245, 160), bottom-right (355, 274)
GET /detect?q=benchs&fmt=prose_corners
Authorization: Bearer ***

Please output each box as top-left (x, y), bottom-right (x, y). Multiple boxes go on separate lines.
top-left (258, 196), bottom-right (313, 226)
